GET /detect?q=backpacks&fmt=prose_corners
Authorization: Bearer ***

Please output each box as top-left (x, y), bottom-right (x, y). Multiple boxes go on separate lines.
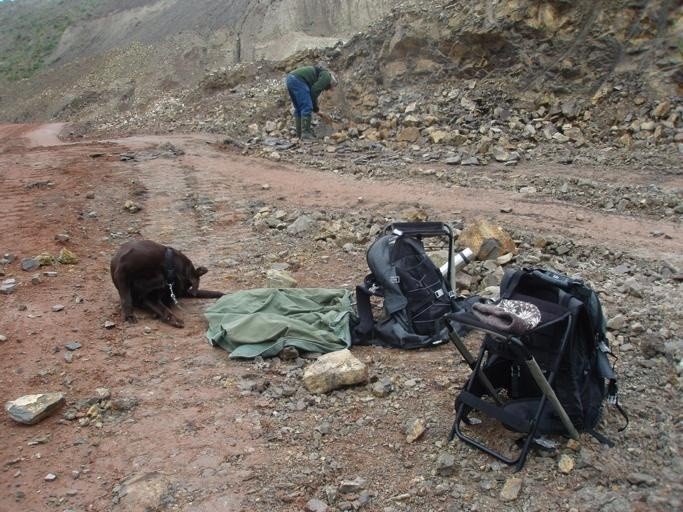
top-left (355, 226), bottom-right (474, 347)
top-left (476, 265), bottom-right (619, 438)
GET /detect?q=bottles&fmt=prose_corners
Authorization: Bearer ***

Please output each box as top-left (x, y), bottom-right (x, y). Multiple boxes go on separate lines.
top-left (438, 247), bottom-right (475, 280)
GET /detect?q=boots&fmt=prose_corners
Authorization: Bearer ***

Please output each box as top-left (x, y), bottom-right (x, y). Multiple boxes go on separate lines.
top-left (293, 117), bottom-right (302, 139)
top-left (299, 116), bottom-right (319, 143)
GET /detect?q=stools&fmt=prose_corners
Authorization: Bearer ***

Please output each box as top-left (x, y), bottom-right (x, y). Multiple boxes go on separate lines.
top-left (385, 224), bottom-right (454, 289)
top-left (441, 294), bottom-right (579, 472)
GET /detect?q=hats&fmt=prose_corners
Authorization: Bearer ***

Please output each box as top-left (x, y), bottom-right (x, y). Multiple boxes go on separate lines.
top-left (471, 297), bottom-right (544, 334)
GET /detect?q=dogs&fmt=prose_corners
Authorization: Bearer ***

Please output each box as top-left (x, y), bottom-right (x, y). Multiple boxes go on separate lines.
top-left (110, 239), bottom-right (227, 329)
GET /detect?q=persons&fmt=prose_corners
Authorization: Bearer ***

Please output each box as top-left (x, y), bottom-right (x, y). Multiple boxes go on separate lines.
top-left (287, 66), bottom-right (340, 144)
